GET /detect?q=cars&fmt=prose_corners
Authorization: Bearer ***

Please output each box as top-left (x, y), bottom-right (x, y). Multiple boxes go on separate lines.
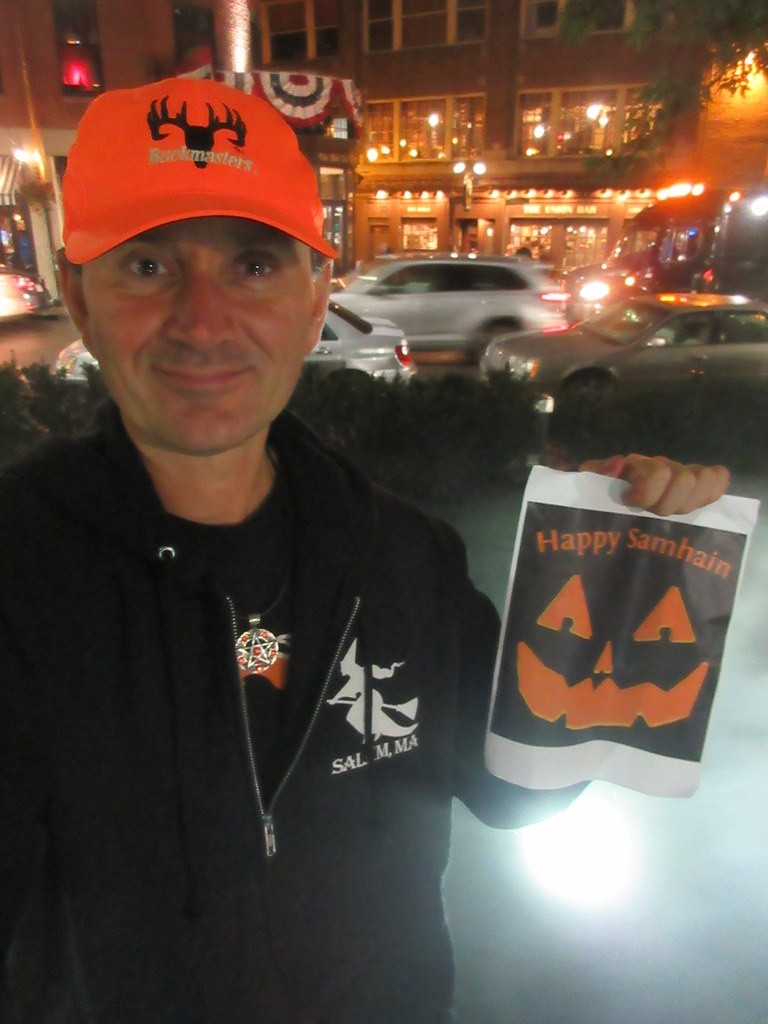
top-left (329, 258), bottom-right (571, 359)
top-left (481, 294), bottom-right (768, 410)
top-left (49, 297), bottom-right (417, 390)
top-left (1, 265), bottom-right (48, 322)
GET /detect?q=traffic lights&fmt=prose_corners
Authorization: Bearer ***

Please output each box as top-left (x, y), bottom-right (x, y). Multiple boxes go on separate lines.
top-left (53, 0), bottom-right (105, 95)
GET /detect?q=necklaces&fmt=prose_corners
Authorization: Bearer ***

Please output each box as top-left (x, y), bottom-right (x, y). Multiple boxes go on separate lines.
top-left (233, 551), bottom-right (294, 675)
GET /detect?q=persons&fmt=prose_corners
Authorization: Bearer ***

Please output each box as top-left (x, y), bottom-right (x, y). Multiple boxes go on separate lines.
top-left (0, 77), bottom-right (732, 1024)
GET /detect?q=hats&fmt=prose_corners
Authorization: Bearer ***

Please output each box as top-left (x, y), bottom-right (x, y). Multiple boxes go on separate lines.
top-left (63, 76), bottom-right (339, 267)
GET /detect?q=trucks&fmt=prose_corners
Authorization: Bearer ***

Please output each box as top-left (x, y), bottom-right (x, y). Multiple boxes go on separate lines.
top-left (565, 184), bottom-right (768, 335)
